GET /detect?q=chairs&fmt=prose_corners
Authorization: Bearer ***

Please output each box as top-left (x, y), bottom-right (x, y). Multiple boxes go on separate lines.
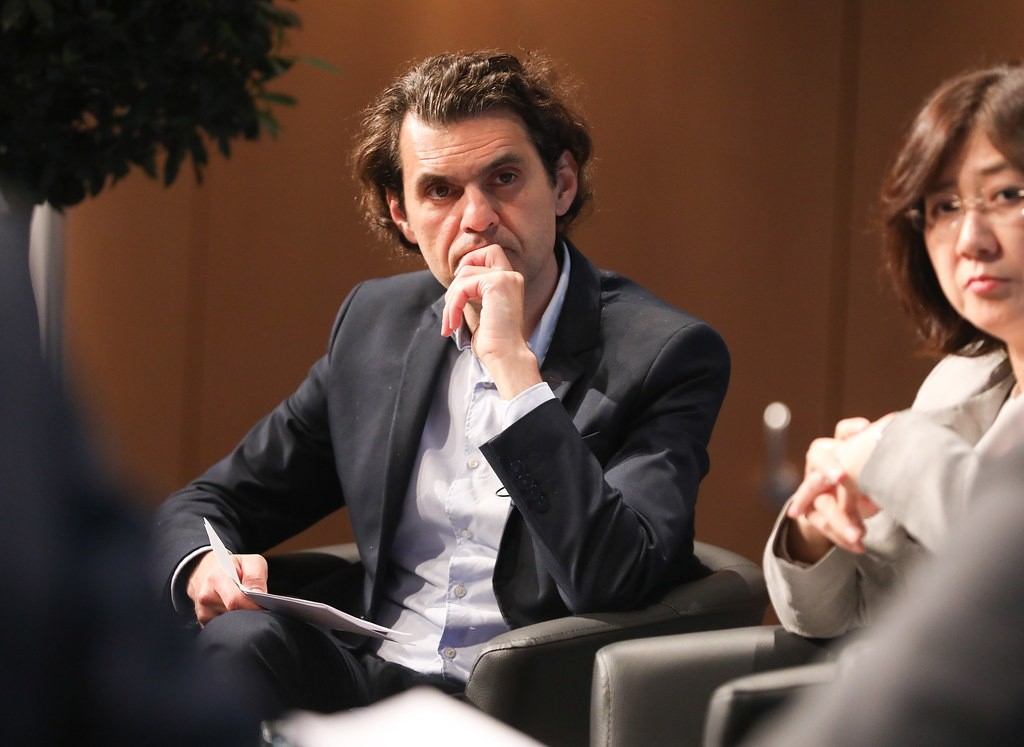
top-left (258, 539), bottom-right (830, 747)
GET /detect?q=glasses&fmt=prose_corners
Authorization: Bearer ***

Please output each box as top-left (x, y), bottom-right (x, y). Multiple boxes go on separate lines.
top-left (905, 184), bottom-right (1024, 231)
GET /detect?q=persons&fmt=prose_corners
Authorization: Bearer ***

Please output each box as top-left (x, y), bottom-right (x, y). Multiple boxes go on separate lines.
top-left (762, 66), bottom-right (1024, 747)
top-left (152, 49), bottom-right (730, 747)
